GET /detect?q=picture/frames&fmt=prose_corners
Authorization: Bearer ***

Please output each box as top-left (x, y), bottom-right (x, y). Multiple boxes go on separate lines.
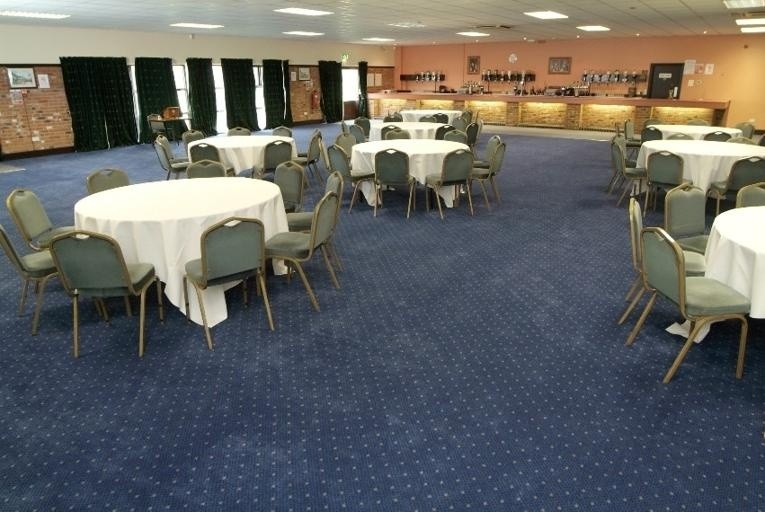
top-left (298, 67), bottom-right (311, 81)
top-left (548, 55), bottom-right (571, 76)
top-left (4, 65), bottom-right (39, 92)
top-left (467, 54), bottom-right (480, 76)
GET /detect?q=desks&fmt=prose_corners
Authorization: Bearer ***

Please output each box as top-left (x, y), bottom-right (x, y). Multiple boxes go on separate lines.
top-left (73, 175), bottom-right (292, 323)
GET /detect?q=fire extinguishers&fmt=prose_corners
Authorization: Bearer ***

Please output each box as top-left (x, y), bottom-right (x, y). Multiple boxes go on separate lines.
top-left (312, 89), bottom-right (320, 109)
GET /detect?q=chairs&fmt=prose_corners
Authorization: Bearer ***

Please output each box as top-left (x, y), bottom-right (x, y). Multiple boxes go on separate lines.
top-left (183, 217), bottom-right (275, 350)
top-left (148, 104), bottom-right (509, 219)
top-left (49, 232), bottom-right (163, 358)
top-left (606, 115), bottom-right (765, 386)
top-left (4, 187), bottom-right (76, 291)
top-left (84, 163), bottom-right (344, 313)
top-left (0, 227), bottom-right (103, 335)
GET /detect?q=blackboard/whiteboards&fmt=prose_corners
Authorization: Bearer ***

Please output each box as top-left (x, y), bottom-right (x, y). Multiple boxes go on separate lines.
top-left (548, 56), bottom-right (572, 74)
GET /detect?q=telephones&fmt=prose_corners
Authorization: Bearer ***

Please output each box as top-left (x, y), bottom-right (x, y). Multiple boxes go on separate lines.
top-left (564, 88), bottom-right (574, 96)
